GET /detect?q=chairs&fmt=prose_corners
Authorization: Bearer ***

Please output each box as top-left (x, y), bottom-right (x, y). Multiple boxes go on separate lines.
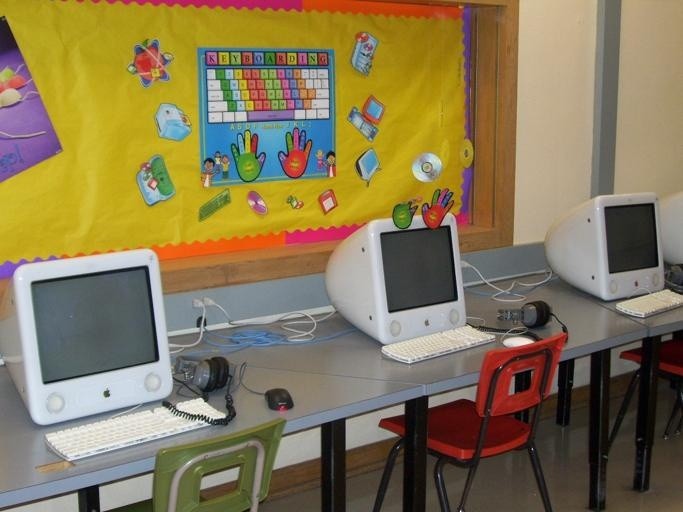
top-left (374, 333), bottom-right (552, 512)
top-left (609, 334), bottom-right (683, 450)
top-left (107, 421), bottom-right (285, 511)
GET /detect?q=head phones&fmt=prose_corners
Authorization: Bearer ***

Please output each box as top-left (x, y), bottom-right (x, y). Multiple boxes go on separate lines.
top-left (175, 355), bottom-right (235, 392)
top-left (496, 300), bottom-right (552, 329)
top-left (664, 266), bottom-right (683, 292)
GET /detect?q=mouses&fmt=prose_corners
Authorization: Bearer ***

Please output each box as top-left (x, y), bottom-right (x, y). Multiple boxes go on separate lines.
top-left (265, 388), bottom-right (294, 411)
top-left (503, 337), bottom-right (534, 348)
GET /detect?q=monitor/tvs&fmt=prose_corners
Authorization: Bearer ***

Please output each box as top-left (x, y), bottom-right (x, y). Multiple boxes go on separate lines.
top-left (0, 248), bottom-right (173, 426)
top-left (323, 212), bottom-right (466, 345)
top-left (543, 192), bottom-right (665, 301)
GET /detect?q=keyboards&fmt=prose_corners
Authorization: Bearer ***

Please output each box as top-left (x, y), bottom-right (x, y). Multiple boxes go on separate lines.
top-left (44, 398), bottom-right (227, 462)
top-left (615, 289), bottom-right (683, 318)
top-left (381, 325), bottom-right (496, 364)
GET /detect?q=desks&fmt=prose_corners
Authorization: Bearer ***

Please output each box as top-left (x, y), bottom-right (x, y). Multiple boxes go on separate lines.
top-left (0, 364), bottom-right (425, 512)
top-left (548, 270), bottom-right (683, 493)
top-left (162, 289), bottom-right (651, 512)
